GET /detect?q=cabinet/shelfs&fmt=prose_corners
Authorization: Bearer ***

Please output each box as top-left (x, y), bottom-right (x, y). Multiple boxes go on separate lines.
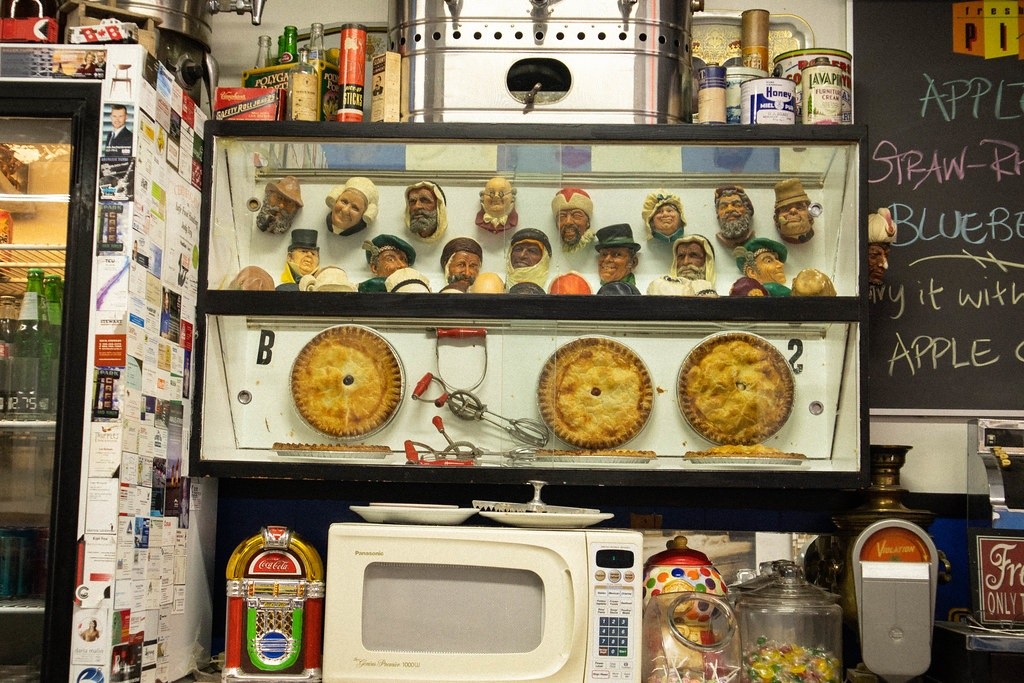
top-left (181, 119), bottom-right (873, 493)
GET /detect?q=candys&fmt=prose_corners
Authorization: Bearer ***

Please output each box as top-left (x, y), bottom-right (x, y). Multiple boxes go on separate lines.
top-left (742, 637), bottom-right (839, 683)
top-left (646, 654), bottom-right (740, 683)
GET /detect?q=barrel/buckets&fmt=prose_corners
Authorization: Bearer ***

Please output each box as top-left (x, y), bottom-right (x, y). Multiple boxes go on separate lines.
top-left (726, 46), bottom-right (852, 127)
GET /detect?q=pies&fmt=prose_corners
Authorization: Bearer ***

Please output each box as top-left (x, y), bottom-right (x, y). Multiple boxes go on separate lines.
top-left (679, 333), bottom-right (793, 446)
top-left (536, 450), bottom-right (658, 459)
top-left (685, 444), bottom-right (806, 459)
top-left (539, 338), bottom-right (653, 449)
top-left (273, 443), bottom-right (391, 452)
top-left (291, 325), bottom-right (401, 438)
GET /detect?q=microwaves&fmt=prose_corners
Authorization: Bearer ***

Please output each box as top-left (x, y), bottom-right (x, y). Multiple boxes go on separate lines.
top-left (322, 523), bottom-right (642, 683)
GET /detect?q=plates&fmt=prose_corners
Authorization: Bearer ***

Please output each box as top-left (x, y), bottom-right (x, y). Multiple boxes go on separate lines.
top-left (681, 463), bottom-right (812, 470)
top-left (350, 505), bottom-right (479, 525)
top-left (480, 512), bottom-right (613, 528)
top-left (270, 454), bottom-right (391, 465)
top-left (533, 462), bottom-right (651, 468)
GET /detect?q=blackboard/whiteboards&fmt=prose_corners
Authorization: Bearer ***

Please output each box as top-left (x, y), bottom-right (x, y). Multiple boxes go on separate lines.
top-left (844, 0), bottom-right (1023, 417)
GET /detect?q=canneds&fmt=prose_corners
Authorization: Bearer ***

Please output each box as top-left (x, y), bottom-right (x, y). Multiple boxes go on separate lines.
top-left (694, 8), bottom-right (853, 126)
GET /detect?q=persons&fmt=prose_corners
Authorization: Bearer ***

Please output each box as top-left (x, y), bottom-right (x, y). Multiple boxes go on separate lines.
top-left (159, 293), bottom-right (171, 339)
top-left (281, 229), bottom-right (320, 283)
top-left (551, 188), bottom-right (595, 252)
top-left (183, 356), bottom-right (189, 399)
top-left (132, 240), bottom-right (138, 261)
top-left (77, 50), bottom-right (106, 73)
top-left (106, 105), bottom-right (133, 145)
top-left (733, 237), bottom-right (791, 296)
top-left (256, 176), bottom-right (304, 234)
top-left (404, 181), bottom-right (448, 243)
top-left (714, 185), bottom-right (755, 246)
top-left (773, 178), bottom-right (815, 242)
top-left (475, 177), bottom-right (519, 234)
top-left (171, 459), bottom-right (179, 484)
top-left (594, 224), bottom-right (641, 295)
top-left (362, 234), bottom-right (416, 276)
top-left (440, 237), bottom-right (482, 287)
top-left (642, 189), bottom-right (687, 244)
top-left (868, 208), bottom-right (897, 285)
top-left (505, 228), bottom-right (553, 285)
top-left (670, 234), bottom-right (716, 288)
top-left (325, 177), bottom-right (379, 236)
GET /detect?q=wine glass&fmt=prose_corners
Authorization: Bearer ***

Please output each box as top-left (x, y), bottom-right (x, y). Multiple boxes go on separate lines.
top-left (525, 480), bottom-right (549, 513)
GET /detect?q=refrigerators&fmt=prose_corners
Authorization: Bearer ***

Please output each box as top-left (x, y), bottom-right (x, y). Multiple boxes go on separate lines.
top-left (0, 43), bottom-right (212, 682)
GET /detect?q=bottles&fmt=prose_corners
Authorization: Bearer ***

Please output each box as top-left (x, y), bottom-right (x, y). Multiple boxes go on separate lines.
top-left (285, 52), bottom-right (319, 121)
top-left (642, 590), bottom-right (742, 683)
top-left (728, 560), bottom-right (843, 683)
top-left (42, 275), bottom-right (63, 414)
top-left (255, 23), bottom-right (324, 69)
top-left (0, 295), bottom-right (16, 412)
top-left (642, 536), bottom-right (729, 613)
top-left (16, 269), bottom-right (50, 413)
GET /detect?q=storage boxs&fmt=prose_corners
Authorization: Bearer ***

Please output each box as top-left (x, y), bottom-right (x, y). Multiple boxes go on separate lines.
top-left (212, 86), bottom-right (287, 120)
top-left (240, 58), bottom-right (340, 121)
top-left (66, 23), bottom-right (140, 43)
top-left (370, 51), bottom-right (402, 122)
top-left (59, 0), bottom-right (163, 58)
